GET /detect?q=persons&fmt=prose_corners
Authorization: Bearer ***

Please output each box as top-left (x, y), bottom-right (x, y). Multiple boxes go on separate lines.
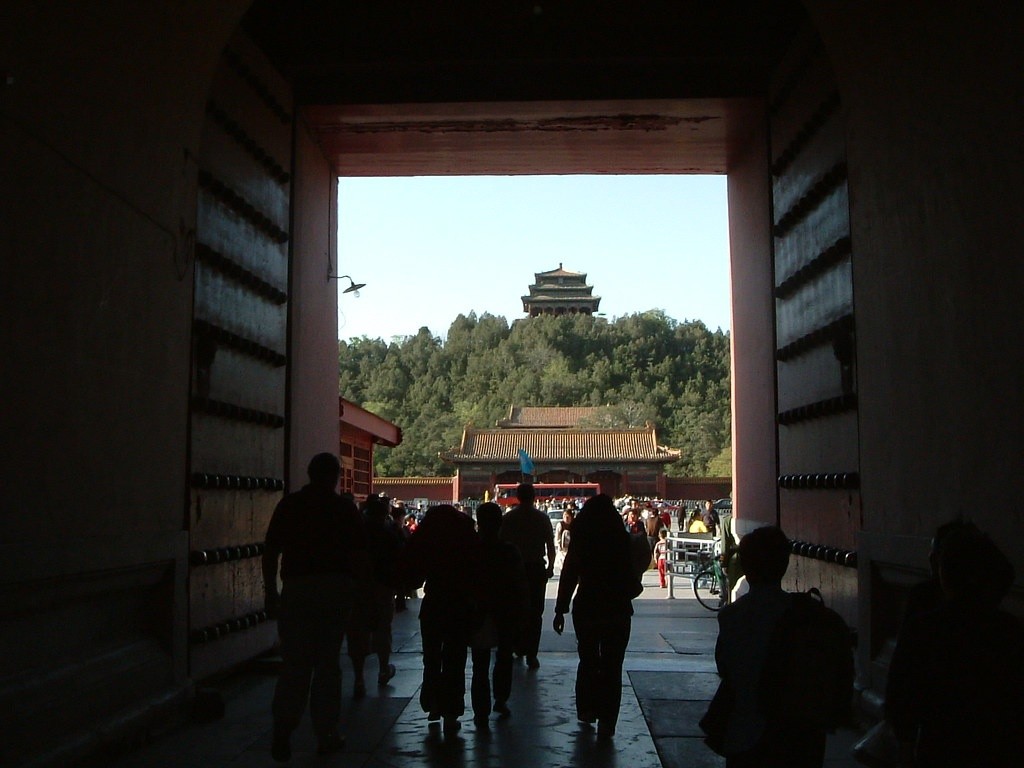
top-left (886, 520), bottom-right (1024, 768)
top-left (259, 450), bottom-right (722, 764)
top-left (715, 526), bottom-right (854, 768)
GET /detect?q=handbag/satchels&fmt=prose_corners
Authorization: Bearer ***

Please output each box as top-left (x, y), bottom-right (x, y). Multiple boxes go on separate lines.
top-left (847, 717), bottom-right (916, 768)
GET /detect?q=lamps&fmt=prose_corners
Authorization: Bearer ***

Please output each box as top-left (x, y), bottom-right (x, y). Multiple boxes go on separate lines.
top-left (327, 272), bottom-right (366, 298)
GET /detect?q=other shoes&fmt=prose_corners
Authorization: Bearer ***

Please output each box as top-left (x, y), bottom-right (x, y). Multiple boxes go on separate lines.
top-left (443, 720), bottom-right (461, 733)
top-left (317, 732), bottom-right (346, 755)
top-left (578, 712), bottom-right (596, 723)
top-left (526, 655), bottom-right (540, 668)
top-left (598, 721), bottom-right (614, 736)
top-left (353, 685), bottom-right (366, 695)
top-left (378, 664), bottom-right (396, 684)
top-left (428, 709), bottom-right (443, 721)
top-left (271, 720), bottom-right (291, 762)
top-left (474, 716), bottom-right (489, 726)
top-left (493, 704), bottom-right (511, 715)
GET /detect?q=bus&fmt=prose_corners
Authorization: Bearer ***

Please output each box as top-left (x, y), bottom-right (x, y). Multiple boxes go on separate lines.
top-left (493, 482), bottom-right (601, 508)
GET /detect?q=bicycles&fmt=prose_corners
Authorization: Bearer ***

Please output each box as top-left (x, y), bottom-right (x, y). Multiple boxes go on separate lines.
top-left (692, 537), bottom-right (747, 611)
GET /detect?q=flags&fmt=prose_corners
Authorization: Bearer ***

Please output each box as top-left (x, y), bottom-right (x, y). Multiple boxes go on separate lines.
top-left (518, 450), bottom-right (536, 475)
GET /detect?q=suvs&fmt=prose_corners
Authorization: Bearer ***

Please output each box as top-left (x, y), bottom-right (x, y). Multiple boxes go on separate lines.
top-left (643, 500), bottom-right (680, 510)
top-left (712, 499), bottom-right (732, 510)
